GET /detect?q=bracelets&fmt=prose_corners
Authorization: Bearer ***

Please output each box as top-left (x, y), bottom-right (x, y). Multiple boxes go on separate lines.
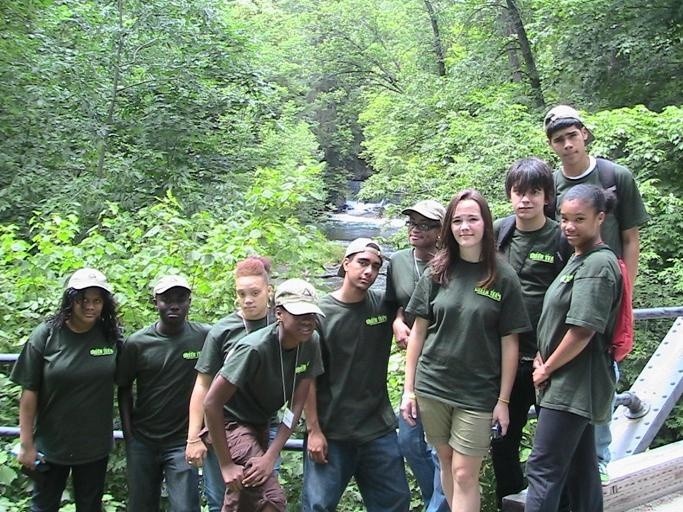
top-left (402, 394), bottom-right (416, 399)
top-left (497, 398), bottom-right (509, 404)
top-left (186, 439), bottom-right (201, 443)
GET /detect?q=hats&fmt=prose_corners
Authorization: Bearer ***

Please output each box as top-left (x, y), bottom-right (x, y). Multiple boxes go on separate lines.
top-left (339, 238), bottom-right (384, 278)
top-left (544, 104), bottom-right (595, 146)
top-left (155, 274), bottom-right (192, 299)
top-left (69, 268), bottom-right (110, 294)
top-left (275, 277), bottom-right (326, 319)
top-left (402, 199), bottom-right (447, 226)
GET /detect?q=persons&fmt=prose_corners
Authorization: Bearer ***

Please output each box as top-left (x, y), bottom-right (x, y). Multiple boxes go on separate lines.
top-left (400, 191), bottom-right (532, 512)
top-left (543, 104), bottom-right (650, 486)
top-left (525, 183), bottom-right (623, 512)
top-left (8, 267), bottom-right (121, 512)
top-left (301, 238), bottom-right (411, 512)
top-left (185, 256), bottom-right (279, 512)
top-left (203, 278), bottom-right (325, 512)
top-left (387, 199), bottom-right (449, 512)
top-left (493, 157), bottom-right (575, 512)
top-left (116, 274), bottom-right (213, 512)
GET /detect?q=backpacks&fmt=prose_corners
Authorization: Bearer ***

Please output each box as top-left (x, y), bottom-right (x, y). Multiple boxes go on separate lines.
top-left (596, 244), bottom-right (635, 363)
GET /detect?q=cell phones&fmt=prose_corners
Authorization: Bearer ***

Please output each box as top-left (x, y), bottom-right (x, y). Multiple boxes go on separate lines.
top-left (491, 420), bottom-right (502, 440)
top-left (11, 442), bottom-right (44, 466)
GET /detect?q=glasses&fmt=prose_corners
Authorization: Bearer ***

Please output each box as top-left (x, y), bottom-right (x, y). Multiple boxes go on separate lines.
top-left (404, 221), bottom-right (442, 232)
